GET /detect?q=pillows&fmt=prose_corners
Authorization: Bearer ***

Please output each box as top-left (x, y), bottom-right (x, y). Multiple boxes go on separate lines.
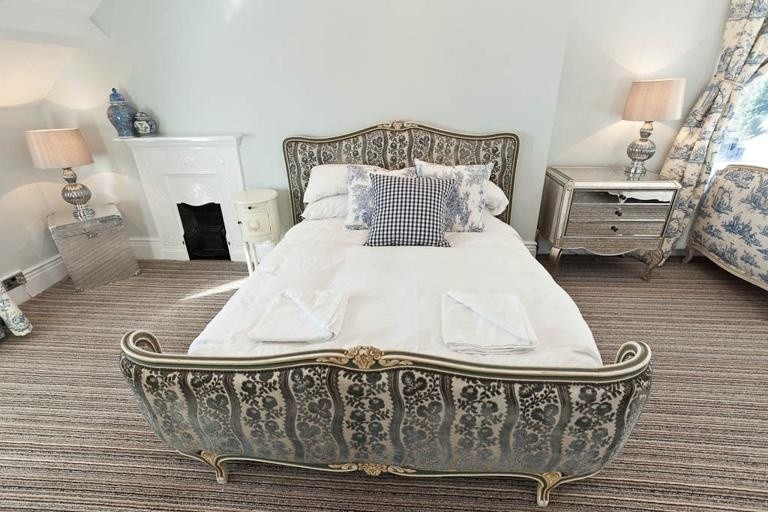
top-left (365, 174), bottom-right (454, 248)
top-left (414, 158), bottom-right (493, 233)
top-left (302, 165), bottom-right (348, 204)
top-left (346, 162), bottom-right (416, 231)
top-left (300, 194), bottom-right (349, 220)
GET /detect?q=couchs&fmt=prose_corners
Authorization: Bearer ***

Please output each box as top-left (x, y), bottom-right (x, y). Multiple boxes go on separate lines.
top-left (683, 163), bottom-right (768, 292)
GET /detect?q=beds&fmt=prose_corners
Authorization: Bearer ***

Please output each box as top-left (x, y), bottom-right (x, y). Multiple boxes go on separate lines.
top-left (119, 118), bottom-right (655, 506)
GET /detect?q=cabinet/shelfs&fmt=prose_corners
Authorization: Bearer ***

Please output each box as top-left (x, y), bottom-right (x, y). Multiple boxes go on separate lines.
top-left (47, 204), bottom-right (141, 292)
top-left (536, 166), bottom-right (682, 282)
top-left (235, 189), bottom-right (280, 276)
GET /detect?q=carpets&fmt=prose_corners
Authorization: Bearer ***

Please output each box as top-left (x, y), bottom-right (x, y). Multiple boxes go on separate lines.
top-left (0, 254), bottom-right (768, 512)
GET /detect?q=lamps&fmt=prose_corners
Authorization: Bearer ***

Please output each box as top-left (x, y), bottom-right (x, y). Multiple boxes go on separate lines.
top-left (24, 128), bottom-right (96, 219)
top-left (621, 77), bottom-right (686, 177)
